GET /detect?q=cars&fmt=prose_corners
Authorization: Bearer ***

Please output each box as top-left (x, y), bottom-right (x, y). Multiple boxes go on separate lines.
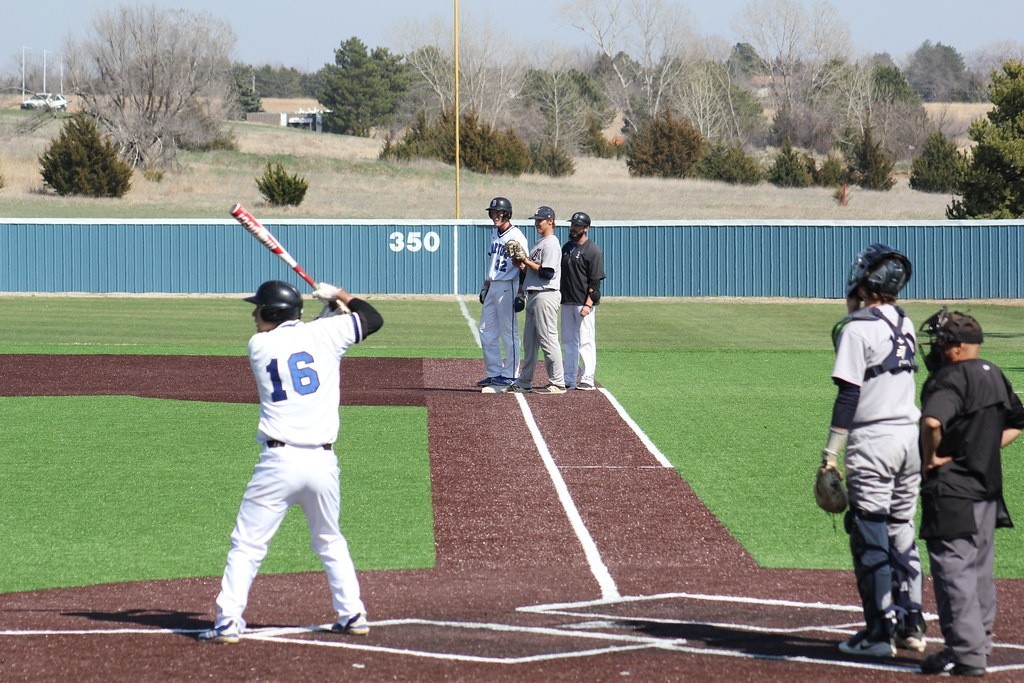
top-left (20, 93), bottom-right (67, 112)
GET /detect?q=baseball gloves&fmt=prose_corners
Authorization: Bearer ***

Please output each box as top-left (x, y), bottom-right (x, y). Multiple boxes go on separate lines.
top-left (504, 239), bottom-right (528, 263)
top-left (814, 467), bottom-right (848, 514)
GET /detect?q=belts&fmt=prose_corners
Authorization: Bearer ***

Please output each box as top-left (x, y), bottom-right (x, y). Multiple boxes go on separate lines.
top-left (526, 290), bottom-right (535, 295)
top-left (267, 440), bottom-right (331, 450)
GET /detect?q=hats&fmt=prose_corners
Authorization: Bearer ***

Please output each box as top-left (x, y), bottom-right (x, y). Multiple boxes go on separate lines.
top-left (937, 318), bottom-right (983, 343)
top-left (528, 206), bottom-right (555, 219)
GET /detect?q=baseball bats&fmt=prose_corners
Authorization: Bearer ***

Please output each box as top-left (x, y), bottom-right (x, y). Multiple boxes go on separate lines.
top-left (228, 201), bottom-right (340, 312)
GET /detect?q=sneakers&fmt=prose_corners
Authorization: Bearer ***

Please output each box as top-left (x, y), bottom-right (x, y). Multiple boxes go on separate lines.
top-left (536, 384), bottom-right (567, 394)
top-left (198, 623), bottom-right (238, 643)
top-left (838, 636), bottom-right (896, 657)
top-left (892, 631), bottom-right (926, 648)
top-left (575, 383), bottom-right (596, 390)
top-left (476, 375), bottom-right (502, 387)
top-left (491, 377), bottom-right (518, 386)
top-left (332, 613), bottom-right (369, 635)
top-left (500, 380), bottom-right (533, 392)
top-left (920, 651), bottom-right (985, 675)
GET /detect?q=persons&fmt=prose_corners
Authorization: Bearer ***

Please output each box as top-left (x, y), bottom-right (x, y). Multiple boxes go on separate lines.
top-left (477, 196), bottom-right (529, 386)
top-left (560, 212), bottom-right (606, 390)
top-left (814, 243), bottom-right (926, 657)
top-left (917, 307), bottom-right (1024, 675)
top-left (501, 207), bottom-right (567, 394)
top-left (198, 280), bottom-right (384, 642)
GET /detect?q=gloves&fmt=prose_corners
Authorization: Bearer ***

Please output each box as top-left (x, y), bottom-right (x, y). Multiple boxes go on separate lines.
top-left (312, 282), bottom-right (342, 301)
top-left (479, 282), bottom-right (489, 303)
top-left (514, 294), bottom-right (524, 313)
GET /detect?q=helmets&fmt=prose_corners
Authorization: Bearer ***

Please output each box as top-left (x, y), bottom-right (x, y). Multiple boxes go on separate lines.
top-left (244, 281), bottom-right (303, 322)
top-left (568, 212), bottom-right (590, 226)
top-left (857, 257), bottom-right (906, 294)
top-left (484, 197), bottom-right (512, 218)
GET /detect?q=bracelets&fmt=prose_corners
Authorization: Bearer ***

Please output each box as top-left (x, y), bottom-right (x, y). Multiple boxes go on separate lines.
top-left (584, 304), bottom-right (591, 308)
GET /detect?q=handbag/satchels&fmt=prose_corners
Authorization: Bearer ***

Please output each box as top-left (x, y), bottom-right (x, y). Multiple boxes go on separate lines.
top-left (920, 491), bottom-right (979, 540)
